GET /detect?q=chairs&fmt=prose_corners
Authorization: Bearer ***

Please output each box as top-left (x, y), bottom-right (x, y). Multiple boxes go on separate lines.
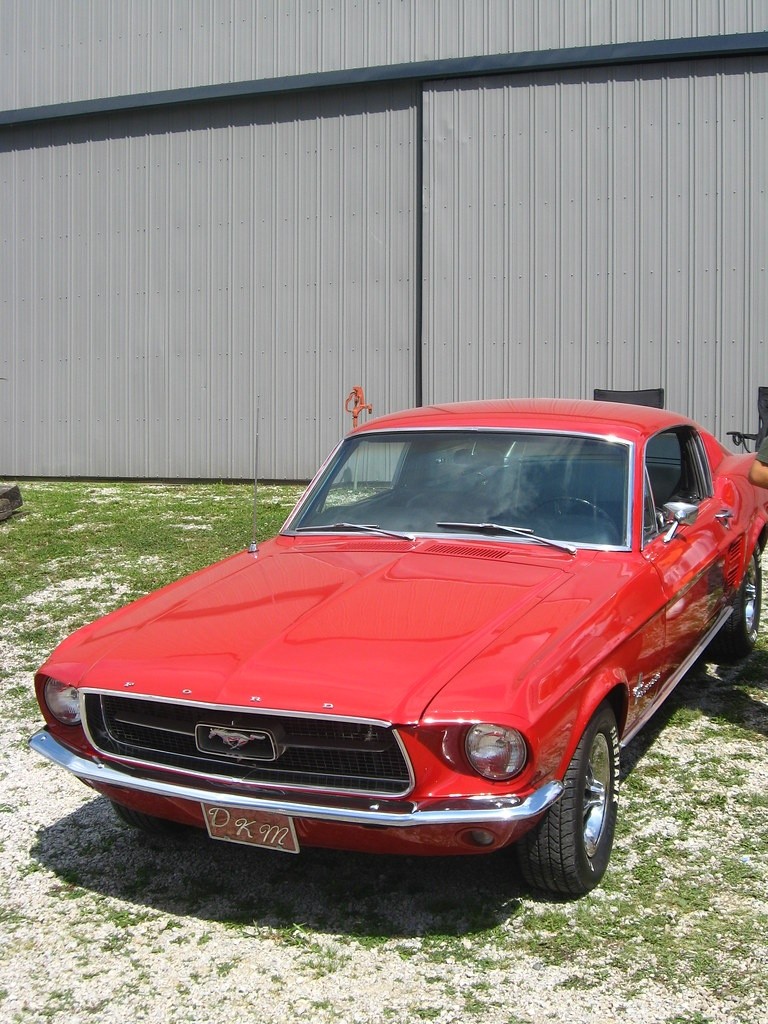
top-left (594, 387), bottom-right (665, 412)
top-left (727, 387), bottom-right (768, 454)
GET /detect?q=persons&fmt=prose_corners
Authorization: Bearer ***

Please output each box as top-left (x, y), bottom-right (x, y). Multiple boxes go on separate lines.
top-left (748, 436), bottom-right (768, 489)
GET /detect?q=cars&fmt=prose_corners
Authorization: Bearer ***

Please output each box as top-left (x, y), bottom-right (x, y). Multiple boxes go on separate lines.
top-left (27, 399), bottom-right (768, 899)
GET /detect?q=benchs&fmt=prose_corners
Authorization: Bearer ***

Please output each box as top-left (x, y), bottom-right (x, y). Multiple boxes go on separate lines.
top-left (484, 460), bottom-right (683, 523)
